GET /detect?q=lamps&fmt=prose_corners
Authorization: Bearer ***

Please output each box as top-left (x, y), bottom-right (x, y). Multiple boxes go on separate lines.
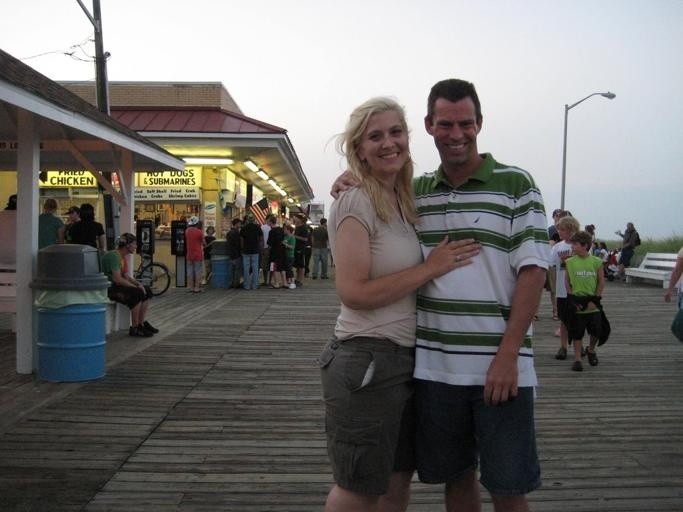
top-left (182, 153), bottom-right (295, 204)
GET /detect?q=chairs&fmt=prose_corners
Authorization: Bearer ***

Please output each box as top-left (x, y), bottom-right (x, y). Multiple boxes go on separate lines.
top-left (0, 264), bottom-right (17, 335)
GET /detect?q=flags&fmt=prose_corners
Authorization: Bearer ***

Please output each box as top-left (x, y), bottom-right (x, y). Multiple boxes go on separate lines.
top-left (248, 197), bottom-right (269, 226)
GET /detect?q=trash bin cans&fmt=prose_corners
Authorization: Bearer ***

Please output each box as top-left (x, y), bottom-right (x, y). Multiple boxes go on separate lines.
top-left (28, 244), bottom-right (112, 382)
top-left (203, 239), bottom-right (234, 289)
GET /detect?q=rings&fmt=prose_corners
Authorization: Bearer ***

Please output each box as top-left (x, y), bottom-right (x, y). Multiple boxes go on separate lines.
top-left (455, 254), bottom-right (460, 262)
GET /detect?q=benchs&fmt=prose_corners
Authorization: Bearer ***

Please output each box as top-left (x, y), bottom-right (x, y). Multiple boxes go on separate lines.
top-left (624, 252), bottom-right (682, 289)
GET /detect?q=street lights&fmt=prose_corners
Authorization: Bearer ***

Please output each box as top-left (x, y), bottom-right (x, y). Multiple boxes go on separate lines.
top-left (559, 91), bottom-right (618, 210)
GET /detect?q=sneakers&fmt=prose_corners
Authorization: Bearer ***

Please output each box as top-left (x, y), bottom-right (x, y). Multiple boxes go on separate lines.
top-left (572, 361), bottom-right (583, 372)
top-left (128, 320), bottom-right (159, 338)
top-left (283, 280), bottom-right (303, 289)
top-left (554, 347), bottom-right (568, 360)
top-left (553, 327), bottom-right (561, 337)
top-left (585, 346), bottom-right (599, 366)
top-left (312, 275), bottom-right (330, 280)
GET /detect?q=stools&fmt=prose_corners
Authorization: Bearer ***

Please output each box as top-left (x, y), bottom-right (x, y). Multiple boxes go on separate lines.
top-left (104, 301), bottom-right (120, 335)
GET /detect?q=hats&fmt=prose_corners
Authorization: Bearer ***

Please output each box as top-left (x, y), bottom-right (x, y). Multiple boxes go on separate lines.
top-left (64, 206), bottom-right (80, 214)
top-left (187, 216), bottom-right (199, 226)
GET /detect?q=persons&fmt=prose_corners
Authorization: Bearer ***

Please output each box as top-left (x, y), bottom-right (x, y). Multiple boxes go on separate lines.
top-left (327, 75), bottom-right (555, 511)
top-left (225, 214), bottom-right (330, 290)
top-left (542, 204), bottom-right (643, 373)
top-left (35, 199), bottom-right (160, 339)
top-left (202, 225), bottom-right (216, 280)
top-left (3, 194), bottom-right (16, 210)
top-left (314, 96), bottom-right (482, 511)
top-left (665, 243), bottom-right (682, 346)
top-left (182, 217), bottom-right (207, 293)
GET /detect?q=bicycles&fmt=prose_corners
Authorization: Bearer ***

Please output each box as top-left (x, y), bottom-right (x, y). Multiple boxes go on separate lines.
top-left (133, 253), bottom-right (171, 297)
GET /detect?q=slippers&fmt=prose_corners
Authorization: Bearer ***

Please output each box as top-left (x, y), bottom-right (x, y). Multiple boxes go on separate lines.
top-left (267, 285), bottom-right (279, 289)
